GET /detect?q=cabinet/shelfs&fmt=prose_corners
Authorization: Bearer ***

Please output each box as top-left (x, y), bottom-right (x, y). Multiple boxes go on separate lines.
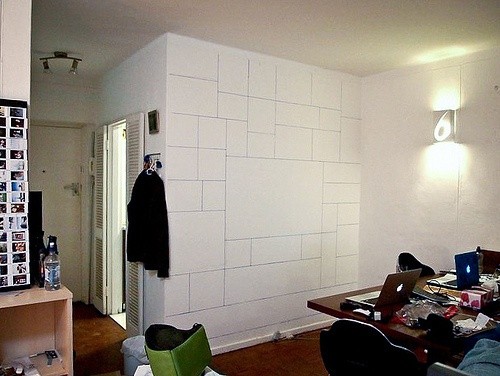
top-left (0, 285), bottom-right (74, 376)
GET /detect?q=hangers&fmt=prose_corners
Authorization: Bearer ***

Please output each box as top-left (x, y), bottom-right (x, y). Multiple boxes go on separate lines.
top-left (145, 158), bottom-right (154, 175)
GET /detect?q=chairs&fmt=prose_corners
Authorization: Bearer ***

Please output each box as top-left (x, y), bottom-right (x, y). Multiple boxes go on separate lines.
top-left (318, 318), bottom-right (422, 376)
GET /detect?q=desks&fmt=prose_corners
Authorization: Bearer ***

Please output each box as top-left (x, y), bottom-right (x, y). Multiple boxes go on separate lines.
top-left (306, 268), bottom-right (500, 351)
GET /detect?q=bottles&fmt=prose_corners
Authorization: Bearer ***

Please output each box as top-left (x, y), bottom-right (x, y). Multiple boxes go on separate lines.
top-left (476, 246), bottom-right (484, 274)
top-left (43, 242), bottom-right (61, 291)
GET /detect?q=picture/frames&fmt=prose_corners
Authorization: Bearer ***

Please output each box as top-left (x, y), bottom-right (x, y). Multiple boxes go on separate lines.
top-left (147, 110), bottom-right (161, 136)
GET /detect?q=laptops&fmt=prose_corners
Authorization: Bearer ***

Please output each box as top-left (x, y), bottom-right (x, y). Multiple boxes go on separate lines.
top-left (426, 251), bottom-right (479, 291)
top-left (344, 268), bottom-right (421, 312)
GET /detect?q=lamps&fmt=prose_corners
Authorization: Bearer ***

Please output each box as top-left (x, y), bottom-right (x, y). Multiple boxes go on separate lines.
top-left (431, 109), bottom-right (457, 145)
top-left (39, 50), bottom-right (82, 75)
